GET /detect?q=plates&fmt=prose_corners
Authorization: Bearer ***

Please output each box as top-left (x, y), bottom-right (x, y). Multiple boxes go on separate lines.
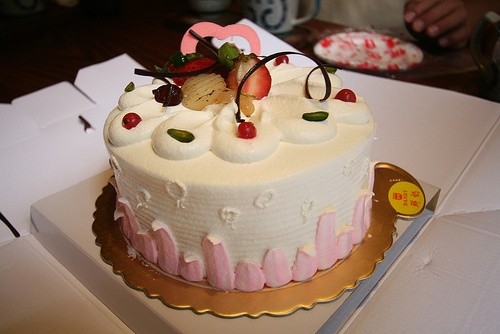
top-left (314, 31), bottom-right (420, 71)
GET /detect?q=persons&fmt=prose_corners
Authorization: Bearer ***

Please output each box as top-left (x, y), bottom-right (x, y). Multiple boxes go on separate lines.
top-left (404, 0), bottom-right (500, 55)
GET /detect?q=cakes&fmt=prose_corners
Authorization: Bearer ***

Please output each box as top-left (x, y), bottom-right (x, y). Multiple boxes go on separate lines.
top-left (103, 23), bottom-right (376, 293)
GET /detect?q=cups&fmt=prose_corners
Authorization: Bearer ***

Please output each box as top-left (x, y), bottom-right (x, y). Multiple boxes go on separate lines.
top-left (188, 0), bottom-right (227, 11)
top-left (244, 0), bottom-right (319, 33)
